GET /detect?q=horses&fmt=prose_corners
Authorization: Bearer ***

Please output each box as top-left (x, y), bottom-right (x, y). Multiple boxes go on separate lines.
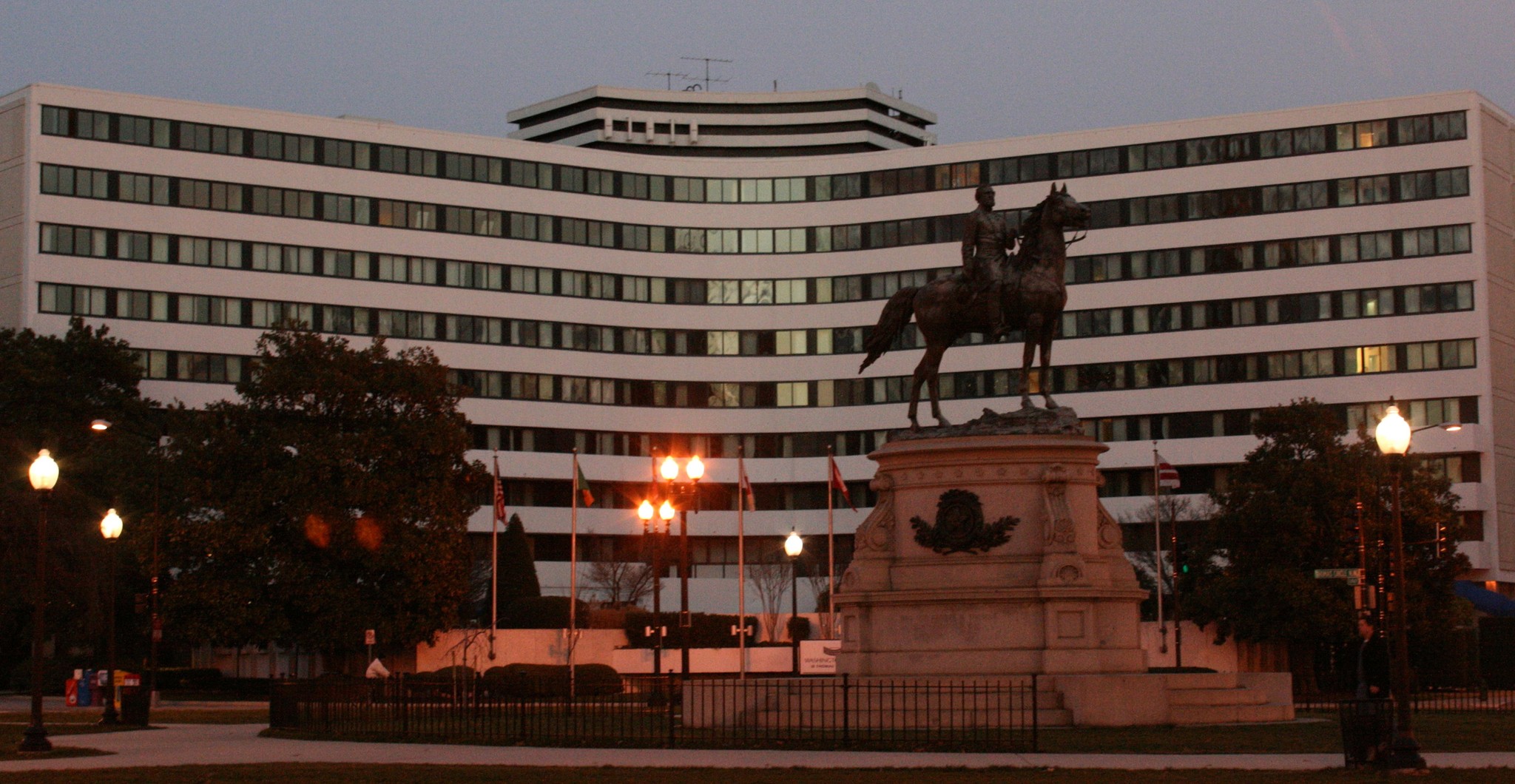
top-left (858, 181), bottom-right (1093, 435)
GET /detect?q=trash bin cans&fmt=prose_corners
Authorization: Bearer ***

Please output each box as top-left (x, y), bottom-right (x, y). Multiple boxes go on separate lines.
top-left (1339, 699), bottom-right (1394, 771)
top-left (119, 685), bottom-right (142, 724)
top-left (269, 683), bottom-right (299, 727)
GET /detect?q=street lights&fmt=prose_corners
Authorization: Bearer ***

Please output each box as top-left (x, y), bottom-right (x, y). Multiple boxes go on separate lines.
top-left (89, 416), bottom-right (163, 711)
top-left (17, 449), bottom-right (59, 752)
top-left (637, 499), bottom-right (675, 707)
top-left (784, 526), bottom-right (804, 678)
top-left (1374, 395), bottom-right (1427, 771)
top-left (98, 507), bottom-right (124, 728)
top-left (1376, 422), bottom-right (1463, 665)
top-left (661, 453), bottom-right (705, 677)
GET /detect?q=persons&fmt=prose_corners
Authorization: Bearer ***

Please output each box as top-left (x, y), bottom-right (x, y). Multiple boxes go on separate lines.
top-left (962, 183), bottom-right (1018, 342)
top-left (1346, 616), bottom-right (1392, 773)
top-left (366, 653), bottom-right (394, 703)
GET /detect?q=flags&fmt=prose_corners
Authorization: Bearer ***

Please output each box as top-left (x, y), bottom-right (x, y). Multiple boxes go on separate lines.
top-left (496, 465), bottom-right (507, 526)
top-left (1157, 456), bottom-right (1180, 493)
top-left (574, 460), bottom-right (594, 507)
top-left (830, 458), bottom-right (856, 510)
top-left (740, 462), bottom-right (755, 511)
